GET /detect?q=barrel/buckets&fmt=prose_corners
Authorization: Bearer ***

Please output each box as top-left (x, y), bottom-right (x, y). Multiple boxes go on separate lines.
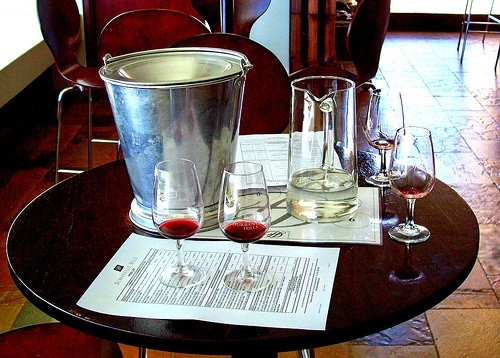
top-left (98, 48), bottom-right (254, 233)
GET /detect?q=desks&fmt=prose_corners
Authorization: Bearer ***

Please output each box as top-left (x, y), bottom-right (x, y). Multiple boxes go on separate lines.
top-left (4, 153), bottom-right (479, 358)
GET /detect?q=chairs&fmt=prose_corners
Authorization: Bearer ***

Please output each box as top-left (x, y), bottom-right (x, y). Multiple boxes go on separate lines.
top-left (0, 322), bottom-right (124, 358)
top-left (37, 0), bottom-right (392, 187)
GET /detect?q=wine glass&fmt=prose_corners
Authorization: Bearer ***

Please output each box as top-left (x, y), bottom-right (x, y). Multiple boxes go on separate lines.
top-left (218, 160), bottom-right (274, 293)
top-left (364, 89), bottom-right (405, 187)
top-left (151, 158), bottom-right (207, 289)
top-left (388, 126), bottom-right (436, 244)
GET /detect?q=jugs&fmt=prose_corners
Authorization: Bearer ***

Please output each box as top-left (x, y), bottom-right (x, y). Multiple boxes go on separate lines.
top-left (287, 73), bottom-right (358, 222)
